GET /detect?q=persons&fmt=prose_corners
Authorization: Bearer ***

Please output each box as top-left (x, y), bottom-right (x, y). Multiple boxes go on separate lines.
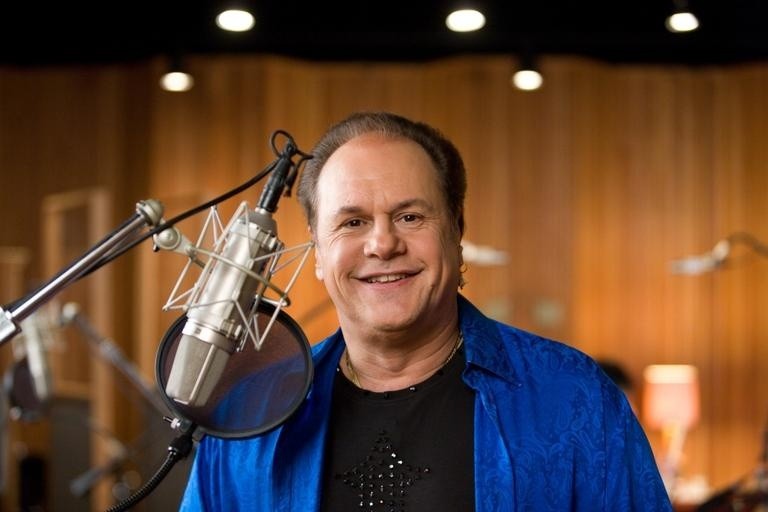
top-left (177, 110), bottom-right (675, 511)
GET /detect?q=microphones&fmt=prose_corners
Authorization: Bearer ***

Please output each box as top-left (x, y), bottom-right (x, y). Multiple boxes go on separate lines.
top-left (164, 157), bottom-right (291, 409)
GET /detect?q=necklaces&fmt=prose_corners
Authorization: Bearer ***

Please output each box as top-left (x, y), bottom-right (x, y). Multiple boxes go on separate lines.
top-left (342, 331), bottom-right (463, 389)
